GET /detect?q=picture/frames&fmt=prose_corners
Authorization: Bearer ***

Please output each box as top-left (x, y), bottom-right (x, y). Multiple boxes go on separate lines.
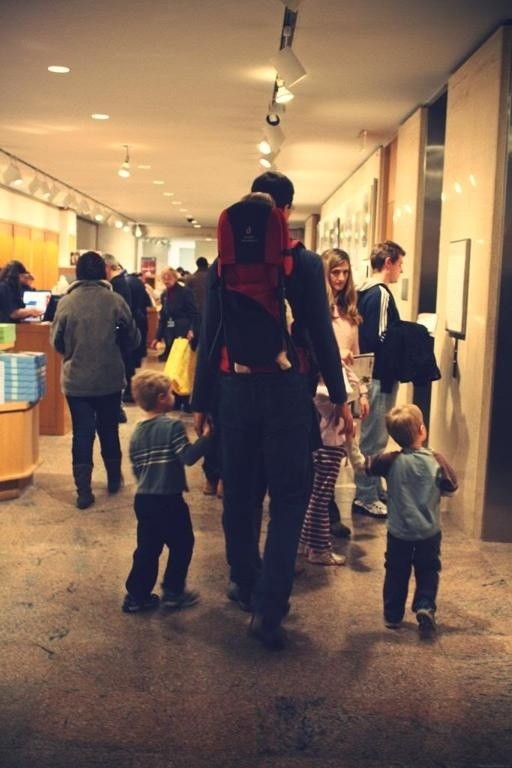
top-left (328, 216), bottom-right (340, 250)
top-left (444, 238), bottom-right (472, 340)
top-left (356, 179), bottom-right (378, 261)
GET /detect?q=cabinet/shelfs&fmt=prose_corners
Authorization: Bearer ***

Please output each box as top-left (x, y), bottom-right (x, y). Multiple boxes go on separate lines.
top-left (12, 320), bottom-right (73, 435)
top-left (145, 307), bottom-right (160, 349)
top-left (1, 396), bottom-right (45, 501)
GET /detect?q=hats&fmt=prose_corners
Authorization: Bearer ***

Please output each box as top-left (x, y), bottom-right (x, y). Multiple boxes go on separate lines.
top-left (76, 252), bottom-right (105, 280)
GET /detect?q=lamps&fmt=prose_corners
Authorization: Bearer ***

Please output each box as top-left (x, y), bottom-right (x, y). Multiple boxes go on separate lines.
top-left (1, 150), bottom-right (144, 238)
top-left (117, 145), bottom-right (133, 177)
top-left (259, 2), bottom-right (307, 177)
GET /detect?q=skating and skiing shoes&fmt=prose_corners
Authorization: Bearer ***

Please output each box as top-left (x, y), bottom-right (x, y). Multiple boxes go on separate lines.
top-left (228, 582), bottom-right (251, 610)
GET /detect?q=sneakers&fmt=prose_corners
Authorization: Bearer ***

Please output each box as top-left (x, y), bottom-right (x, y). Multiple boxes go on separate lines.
top-left (204, 479), bottom-right (217, 495)
top-left (217, 479), bottom-right (224, 497)
top-left (248, 616), bottom-right (287, 651)
top-left (416, 608), bottom-right (435, 632)
top-left (330, 522), bottom-right (350, 536)
top-left (183, 404), bottom-right (192, 413)
top-left (163, 590), bottom-right (201, 607)
top-left (122, 592), bottom-right (158, 611)
top-left (306, 550), bottom-right (347, 565)
top-left (354, 499), bottom-right (388, 517)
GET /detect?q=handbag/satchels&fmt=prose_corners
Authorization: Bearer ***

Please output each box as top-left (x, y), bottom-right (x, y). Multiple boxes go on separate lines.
top-left (164, 337), bottom-right (197, 396)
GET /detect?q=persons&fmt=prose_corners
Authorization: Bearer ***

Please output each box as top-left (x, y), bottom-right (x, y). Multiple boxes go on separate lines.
top-left (121, 367), bottom-right (214, 615)
top-left (340, 401), bottom-right (461, 638)
top-left (1, 237), bottom-right (443, 567)
top-left (187, 168), bottom-right (356, 647)
top-left (231, 189), bottom-right (295, 375)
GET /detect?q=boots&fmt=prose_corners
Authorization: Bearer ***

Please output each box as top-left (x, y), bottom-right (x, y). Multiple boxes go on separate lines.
top-left (101, 452), bottom-right (124, 495)
top-left (74, 464), bottom-right (94, 508)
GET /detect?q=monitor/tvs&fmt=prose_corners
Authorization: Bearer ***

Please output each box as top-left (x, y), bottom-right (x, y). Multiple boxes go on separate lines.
top-left (21, 290), bottom-right (53, 322)
